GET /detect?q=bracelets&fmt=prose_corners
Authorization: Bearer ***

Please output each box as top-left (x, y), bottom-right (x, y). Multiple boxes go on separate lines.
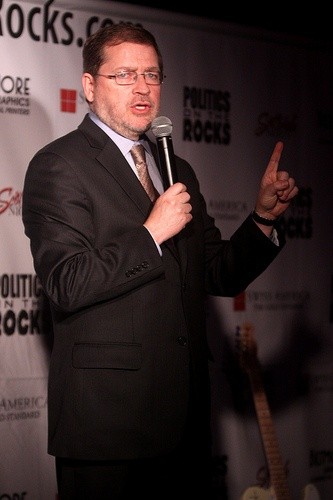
top-left (253, 210), bottom-right (277, 226)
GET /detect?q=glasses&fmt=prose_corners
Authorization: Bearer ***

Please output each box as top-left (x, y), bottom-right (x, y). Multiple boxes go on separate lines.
top-left (92, 71), bottom-right (167, 85)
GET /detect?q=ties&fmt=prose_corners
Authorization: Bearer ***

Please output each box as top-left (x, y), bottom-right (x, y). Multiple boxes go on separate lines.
top-left (130, 144), bottom-right (161, 205)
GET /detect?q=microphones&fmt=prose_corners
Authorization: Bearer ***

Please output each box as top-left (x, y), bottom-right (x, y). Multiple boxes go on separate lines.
top-left (151, 115), bottom-right (178, 191)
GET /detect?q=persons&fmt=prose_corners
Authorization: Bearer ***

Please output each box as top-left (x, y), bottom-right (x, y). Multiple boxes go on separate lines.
top-left (22, 24), bottom-right (298, 500)
